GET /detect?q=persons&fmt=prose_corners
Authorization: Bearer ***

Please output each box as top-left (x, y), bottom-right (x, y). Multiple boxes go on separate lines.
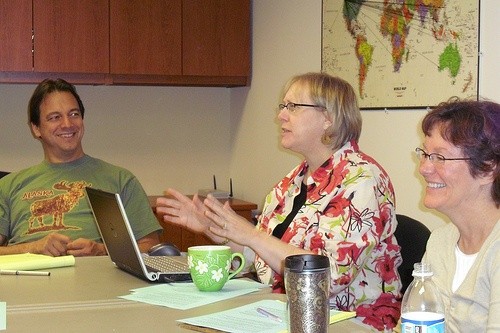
top-left (155, 72), bottom-right (402, 333)
top-left (390, 95), bottom-right (500, 333)
top-left (0, 77), bottom-right (163, 255)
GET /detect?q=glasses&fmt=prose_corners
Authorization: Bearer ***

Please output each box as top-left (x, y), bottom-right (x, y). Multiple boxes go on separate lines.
top-left (279, 102), bottom-right (326, 112)
top-left (415, 147), bottom-right (476, 165)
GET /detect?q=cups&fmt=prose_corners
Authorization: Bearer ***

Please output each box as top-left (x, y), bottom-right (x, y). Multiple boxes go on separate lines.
top-left (187, 245), bottom-right (244, 292)
top-left (284, 253), bottom-right (331, 333)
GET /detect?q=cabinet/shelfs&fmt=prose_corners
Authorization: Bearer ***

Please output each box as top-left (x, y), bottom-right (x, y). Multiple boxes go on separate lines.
top-left (0, 0), bottom-right (254, 88)
top-left (144, 195), bottom-right (257, 252)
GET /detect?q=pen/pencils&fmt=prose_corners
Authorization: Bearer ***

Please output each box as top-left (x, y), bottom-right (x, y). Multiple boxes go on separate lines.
top-left (257, 307), bottom-right (282, 321)
top-left (0, 270), bottom-right (50, 276)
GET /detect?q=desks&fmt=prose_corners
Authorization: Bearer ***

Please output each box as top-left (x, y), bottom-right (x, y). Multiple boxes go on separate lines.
top-left (0, 255), bottom-right (382, 333)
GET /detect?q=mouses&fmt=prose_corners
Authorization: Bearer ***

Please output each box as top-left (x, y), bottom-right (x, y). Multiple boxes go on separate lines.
top-left (148, 242), bottom-right (181, 257)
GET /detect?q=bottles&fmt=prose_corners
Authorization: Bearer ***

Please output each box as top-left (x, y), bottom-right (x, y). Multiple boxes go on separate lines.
top-left (400, 262), bottom-right (445, 333)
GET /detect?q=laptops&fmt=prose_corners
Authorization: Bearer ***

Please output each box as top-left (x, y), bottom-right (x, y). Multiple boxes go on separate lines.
top-left (84, 186), bottom-right (255, 282)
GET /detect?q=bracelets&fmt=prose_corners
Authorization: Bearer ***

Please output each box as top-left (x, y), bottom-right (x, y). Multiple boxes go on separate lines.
top-left (217, 239), bottom-right (229, 245)
